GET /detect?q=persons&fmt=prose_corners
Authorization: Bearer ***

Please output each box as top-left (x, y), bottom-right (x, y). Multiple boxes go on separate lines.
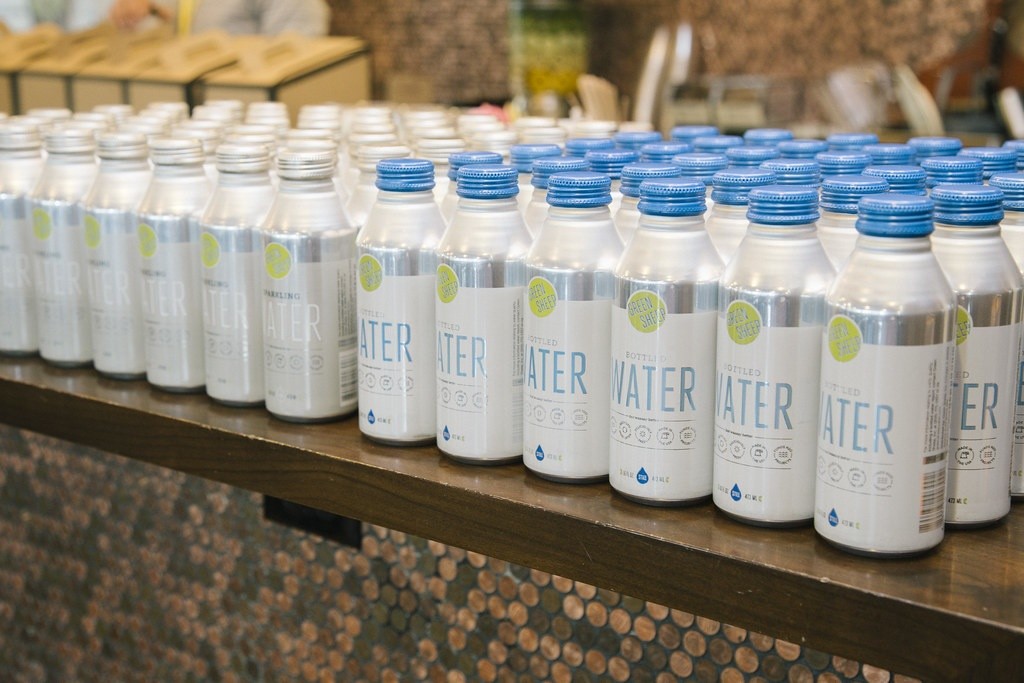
top-left (0, 0), bottom-right (330, 39)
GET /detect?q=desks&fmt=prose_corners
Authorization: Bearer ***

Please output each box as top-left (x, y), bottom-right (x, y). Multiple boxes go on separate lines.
top-left (1, 352), bottom-right (1024, 682)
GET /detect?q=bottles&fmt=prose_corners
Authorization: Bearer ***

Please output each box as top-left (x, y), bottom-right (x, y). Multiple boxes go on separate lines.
top-left (0, 100), bottom-right (1024, 560)
